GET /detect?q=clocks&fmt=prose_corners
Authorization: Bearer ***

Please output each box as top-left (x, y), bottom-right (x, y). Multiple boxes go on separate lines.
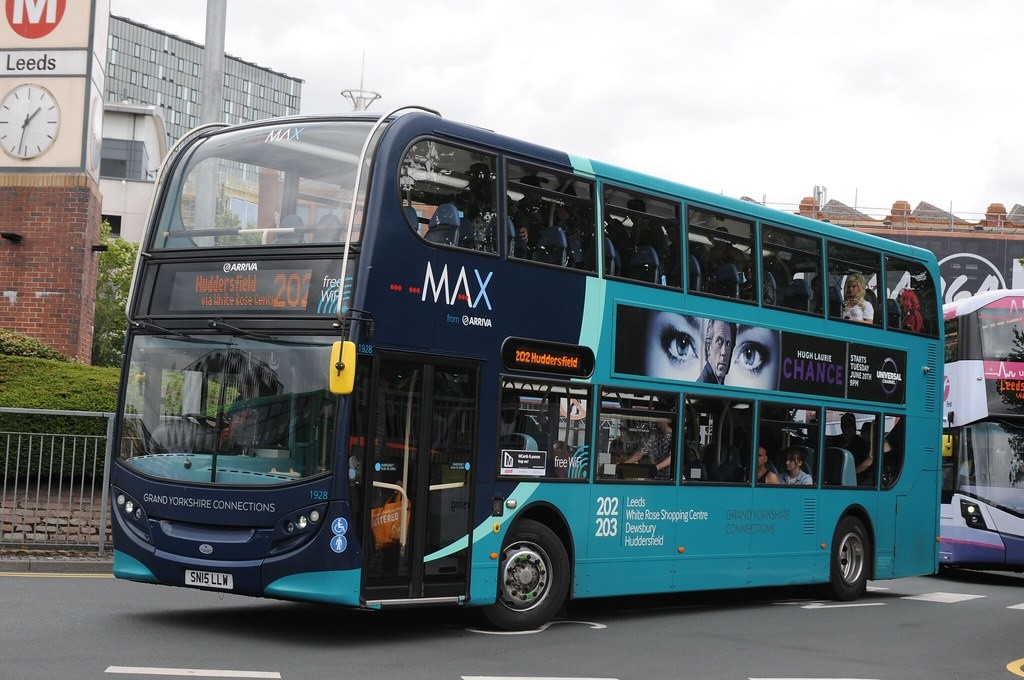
top-left (0, 83), bottom-right (60, 160)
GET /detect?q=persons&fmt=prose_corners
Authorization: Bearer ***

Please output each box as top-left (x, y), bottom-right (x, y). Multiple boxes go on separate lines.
top-left (499, 392), bottom-right (898, 488)
top-left (642, 310), bottom-right (780, 392)
top-left (214, 372), bottom-right (269, 456)
top-left (960, 448), bottom-right (993, 486)
top-left (442, 163), bottom-right (924, 333)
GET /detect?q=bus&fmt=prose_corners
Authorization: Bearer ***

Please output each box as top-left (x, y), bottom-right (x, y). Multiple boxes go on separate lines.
top-left (941, 289), bottom-right (1024, 569)
top-left (110, 104), bottom-right (947, 631)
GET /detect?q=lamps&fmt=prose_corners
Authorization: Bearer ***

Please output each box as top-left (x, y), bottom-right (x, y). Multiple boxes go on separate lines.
top-left (883, 221), bottom-right (892, 229)
top-left (974, 225), bottom-right (983, 232)
top-left (821, 218), bottom-right (830, 222)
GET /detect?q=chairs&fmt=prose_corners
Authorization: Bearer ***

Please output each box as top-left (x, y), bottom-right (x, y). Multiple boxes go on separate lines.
top-left (272, 200), bottom-right (932, 336)
top-left (500, 431), bottom-right (893, 486)
